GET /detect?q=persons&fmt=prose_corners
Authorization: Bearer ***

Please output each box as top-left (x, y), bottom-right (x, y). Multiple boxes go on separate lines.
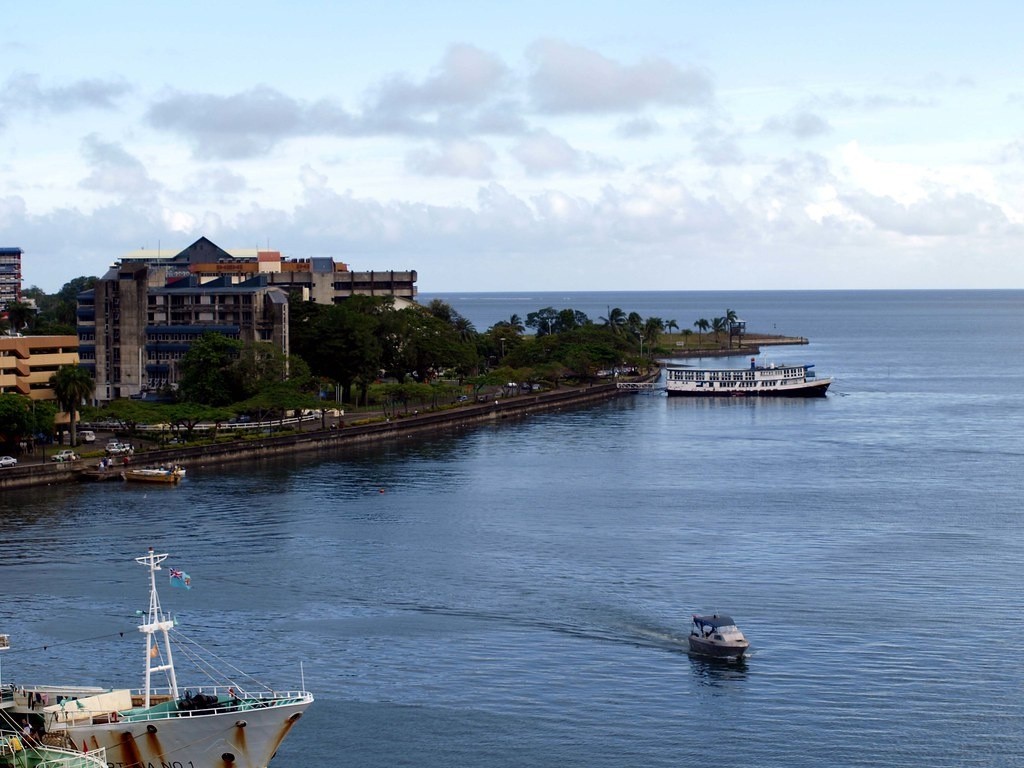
top-left (8, 718), bottom-right (40, 754)
top-left (58, 454), bottom-right (185, 475)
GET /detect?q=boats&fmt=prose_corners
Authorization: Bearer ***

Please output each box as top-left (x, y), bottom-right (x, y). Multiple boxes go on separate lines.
top-left (133, 463), bottom-right (186, 477)
top-left (83, 469), bottom-right (127, 481)
top-left (0, 633), bottom-right (108, 768)
top-left (125, 469), bottom-right (175, 482)
top-left (688, 610), bottom-right (750, 658)
top-left (0, 546), bottom-right (314, 768)
top-left (664, 357), bottom-right (832, 397)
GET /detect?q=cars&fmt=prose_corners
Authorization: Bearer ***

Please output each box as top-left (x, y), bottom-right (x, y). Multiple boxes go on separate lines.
top-left (105, 443), bottom-right (118, 452)
top-left (0, 456), bottom-right (17, 468)
top-left (507, 380), bottom-right (518, 387)
top-left (51, 450), bottom-right (75, 461)
top-left (521, 382), bottom-right (532, 389)
top-left (595, 366), bottom-right (635, 376)
top-left (79, 431), bottom-right (96, 443)
top-left (533, 384), bottom-right (542, 389)
top-left (169, 438), bottom-right (187, 445)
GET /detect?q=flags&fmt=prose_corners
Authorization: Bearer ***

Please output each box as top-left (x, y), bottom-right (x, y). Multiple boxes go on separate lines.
top-left (169, 567), bottom-right (192, 591)
